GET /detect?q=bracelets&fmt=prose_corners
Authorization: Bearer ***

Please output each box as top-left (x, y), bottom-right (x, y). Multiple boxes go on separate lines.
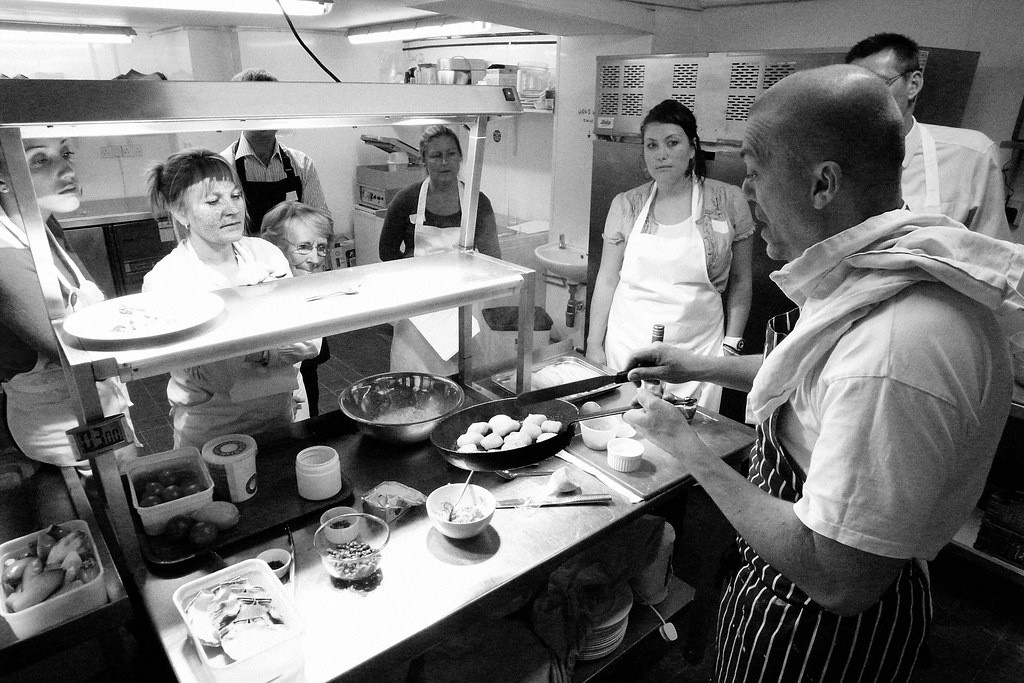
top-left (722, 347), bottom-right (740, 357)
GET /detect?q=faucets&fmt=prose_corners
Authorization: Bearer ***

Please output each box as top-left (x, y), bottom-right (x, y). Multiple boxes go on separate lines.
top-left (558, 233), bottom-right (567, 248)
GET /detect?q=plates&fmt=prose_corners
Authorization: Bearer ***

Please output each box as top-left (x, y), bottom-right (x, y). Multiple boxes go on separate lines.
top-left (63, 293), bottom-right (225, 340)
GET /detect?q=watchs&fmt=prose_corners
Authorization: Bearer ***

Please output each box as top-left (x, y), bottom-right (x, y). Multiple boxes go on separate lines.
top-left (722, 336), bottom-right (745, 352)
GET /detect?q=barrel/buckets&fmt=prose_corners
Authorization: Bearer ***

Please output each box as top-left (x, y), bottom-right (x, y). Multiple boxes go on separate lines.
top-left (202, 434), bottom-right (261, 503)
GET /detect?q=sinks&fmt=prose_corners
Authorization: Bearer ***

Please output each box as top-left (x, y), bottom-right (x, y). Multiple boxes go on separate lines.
top-left (535, 241), bottom-right (587, 283)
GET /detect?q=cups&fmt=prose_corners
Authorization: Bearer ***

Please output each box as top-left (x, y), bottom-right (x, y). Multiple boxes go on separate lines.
top-left (296, 446), bottom-right (341, 499)
top-left (255, 548), bottom-right (290, 578)
top-left (674, 405), bottom-right (697, 426)
top-left (319, 506), bottom-right (359, 544)
top-left (607, 438), bottom-right (645, 472)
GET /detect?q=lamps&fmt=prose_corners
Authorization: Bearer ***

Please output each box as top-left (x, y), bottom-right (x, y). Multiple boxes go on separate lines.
top-left (346, 13), bottom-right (493, 45)
top-left (0, 18), bottom-right (136, 45)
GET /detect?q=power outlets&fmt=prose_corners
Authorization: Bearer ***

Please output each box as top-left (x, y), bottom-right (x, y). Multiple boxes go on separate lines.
top-left (99, 144), bottom-right (144, 158)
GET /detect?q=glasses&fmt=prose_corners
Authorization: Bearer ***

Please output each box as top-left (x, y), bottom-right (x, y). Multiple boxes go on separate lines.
top-left (881, 68), bottom-right (913, 88)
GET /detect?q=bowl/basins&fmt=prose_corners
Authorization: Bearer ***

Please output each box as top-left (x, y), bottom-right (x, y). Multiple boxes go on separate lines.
top-left (312, 513), bottom-right (390, 580)
top-left (426, 483), bottom-right (496, 539)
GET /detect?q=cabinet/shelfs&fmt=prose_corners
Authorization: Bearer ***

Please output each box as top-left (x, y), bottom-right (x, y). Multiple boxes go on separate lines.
top-left (0, 79), bottom-right (537, 581)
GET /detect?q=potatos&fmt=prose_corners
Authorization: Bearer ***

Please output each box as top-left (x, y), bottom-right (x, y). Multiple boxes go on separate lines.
top-left (190, 502), bottom-right (240, 529)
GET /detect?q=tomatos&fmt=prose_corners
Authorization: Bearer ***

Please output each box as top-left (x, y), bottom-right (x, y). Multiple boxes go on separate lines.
top-left (140, 468), bottom-right (218, 546)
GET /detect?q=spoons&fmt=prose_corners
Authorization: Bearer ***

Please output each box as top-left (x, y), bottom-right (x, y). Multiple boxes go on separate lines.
top-left (494, 469), bottom-right (554, 479)
top-left (307, 283), bottom-right (364, 302)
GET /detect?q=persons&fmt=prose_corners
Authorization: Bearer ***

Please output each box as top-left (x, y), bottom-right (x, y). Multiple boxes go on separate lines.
top-left (378, 124), bottom-right (504, 390)
top-left (584, 100), bottom-right (757, 542)
top-left (623, 64), bottom-right (1012, 683)
top-left (845, 32), bottom-right (1013, 243)
top-left (213, 69), bottom-right (333, 417)
top-left (140, 148), bottom-right (326, 454)
top-left (0, 137), bottom-right (143, 479)
top-left (261, 199), bottom-right (334, 423)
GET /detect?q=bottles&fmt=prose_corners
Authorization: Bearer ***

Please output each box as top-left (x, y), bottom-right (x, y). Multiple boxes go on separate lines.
top-left (644, 324), bottom-right (664, 400)
top-left (517, 65), bottom-right (548, 95)
top-left (413, 64), bottom-right (435, 84)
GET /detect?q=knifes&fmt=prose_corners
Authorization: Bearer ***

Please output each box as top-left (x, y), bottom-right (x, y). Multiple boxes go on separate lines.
top-left (516, 370), bottom-right (633, 404)
top-left (495, 494), bottom-right (612, 509)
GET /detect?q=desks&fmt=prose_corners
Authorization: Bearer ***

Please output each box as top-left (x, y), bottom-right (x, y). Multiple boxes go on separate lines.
top-left (51, 196), bottom-right (175, 299)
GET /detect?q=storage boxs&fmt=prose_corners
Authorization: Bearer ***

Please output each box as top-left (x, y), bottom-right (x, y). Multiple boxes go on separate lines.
top-left (125, 446), bottom-right (215, 536)
top-left (1, 519), bottom-right (108, 640)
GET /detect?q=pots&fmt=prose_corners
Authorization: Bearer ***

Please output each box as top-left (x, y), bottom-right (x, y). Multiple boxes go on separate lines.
top-left (429, 397), bottom-right (698, 472)
